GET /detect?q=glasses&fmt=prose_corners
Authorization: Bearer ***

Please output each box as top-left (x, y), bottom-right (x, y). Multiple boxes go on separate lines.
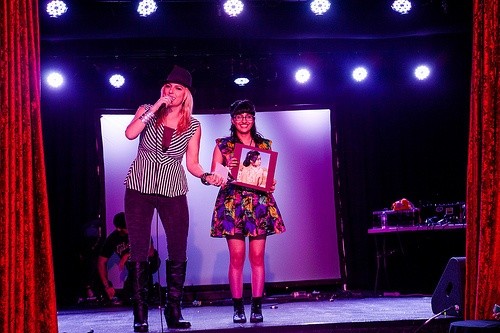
top-left (232, 115), bottom-right (255, 121)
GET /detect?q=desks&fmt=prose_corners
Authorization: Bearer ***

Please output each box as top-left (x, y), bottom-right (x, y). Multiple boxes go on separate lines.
top-left (368, 222), bottom-right (466, 297)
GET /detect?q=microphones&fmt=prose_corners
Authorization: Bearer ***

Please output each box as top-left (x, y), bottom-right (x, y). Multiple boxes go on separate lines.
top-left (157, 103), bottom-right (166, 112)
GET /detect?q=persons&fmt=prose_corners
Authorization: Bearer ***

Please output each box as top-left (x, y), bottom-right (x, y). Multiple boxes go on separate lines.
top-left (98, 212), bottom-right (161, 305)
top-left (210, 99), bottom-right (286, 323)
top-left (124, 64), bottom-right (226, 332)
top-left (239, 151), bottom-right (263, 186)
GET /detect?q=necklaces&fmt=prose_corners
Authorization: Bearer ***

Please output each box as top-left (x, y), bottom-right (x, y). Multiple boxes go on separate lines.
top-left (250, 139), bottom-right (253, 145)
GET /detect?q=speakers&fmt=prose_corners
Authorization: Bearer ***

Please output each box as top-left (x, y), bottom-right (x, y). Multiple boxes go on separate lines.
top-left (431, 257), bottom-right (466, 315)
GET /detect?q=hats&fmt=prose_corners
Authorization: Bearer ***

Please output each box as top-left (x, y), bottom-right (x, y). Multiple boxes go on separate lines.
top-left (113, 212), bottom-right (127, 229)
top-left (166, 65), bottom-right (191, 88)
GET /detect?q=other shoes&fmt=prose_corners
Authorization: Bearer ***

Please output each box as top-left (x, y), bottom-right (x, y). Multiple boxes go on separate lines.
top-left (111, 297), bottom-right (123, 305)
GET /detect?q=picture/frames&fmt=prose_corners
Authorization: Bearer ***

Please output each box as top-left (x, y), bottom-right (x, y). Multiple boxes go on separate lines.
top-left (229, 143), bottom-right (278, 191)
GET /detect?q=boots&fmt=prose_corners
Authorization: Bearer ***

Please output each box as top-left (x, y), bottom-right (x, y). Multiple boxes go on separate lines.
top-left (250, 298), bottom-right (264, 323)
top-left (129, 260), bottom-right (151, 332)
top-left (164, 257), bottom-right (191, 329)
top-left (232, 297), bottom-right (247, 323)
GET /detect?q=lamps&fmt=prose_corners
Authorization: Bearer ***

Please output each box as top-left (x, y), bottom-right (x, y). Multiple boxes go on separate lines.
top-left (230, 48), bottom-right (255, 87)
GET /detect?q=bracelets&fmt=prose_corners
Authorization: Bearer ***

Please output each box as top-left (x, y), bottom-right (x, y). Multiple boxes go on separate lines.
top-left (201, 173), bottom-right (213, 186)
top-left (139, 108), bottom-right (156, 126)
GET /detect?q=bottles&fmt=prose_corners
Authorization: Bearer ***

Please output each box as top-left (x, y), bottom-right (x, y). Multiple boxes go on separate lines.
top-left (381, 212), bottom-right (387, 228)
